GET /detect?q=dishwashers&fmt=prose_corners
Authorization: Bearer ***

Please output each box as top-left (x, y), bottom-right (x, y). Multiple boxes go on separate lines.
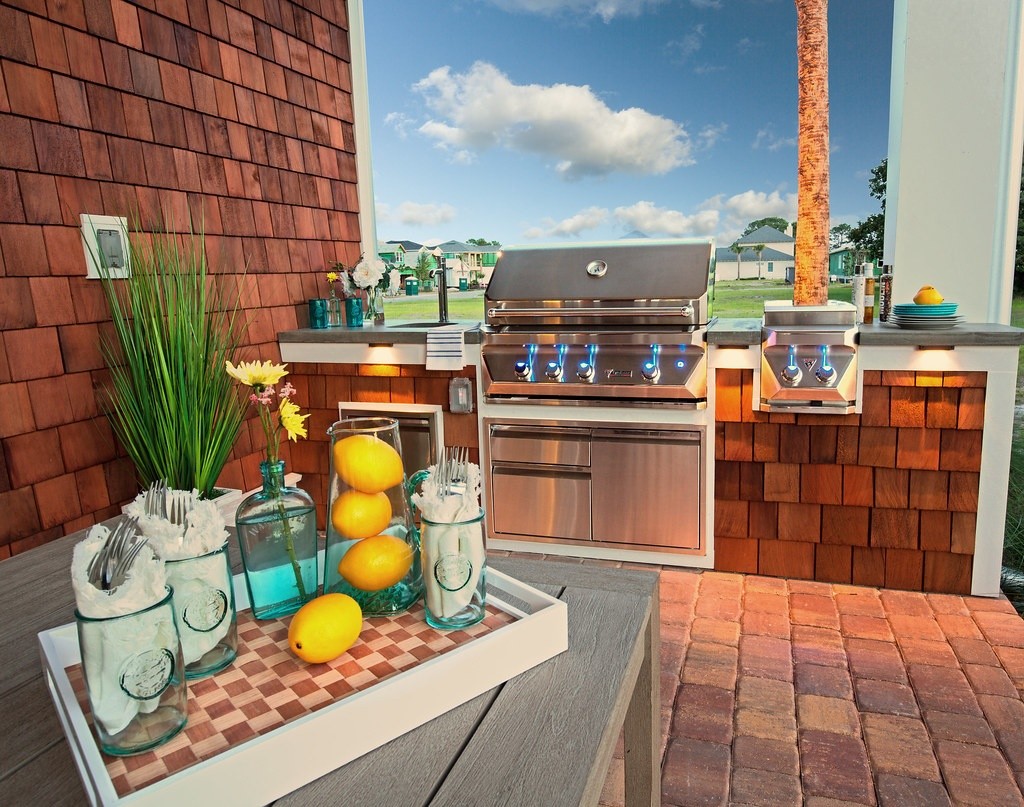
top-left (339, 405), bottom-right (436, 526)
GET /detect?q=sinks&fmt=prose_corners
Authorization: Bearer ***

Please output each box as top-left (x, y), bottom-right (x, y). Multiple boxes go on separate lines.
top-left (387, 322), bottom-right (459, 328)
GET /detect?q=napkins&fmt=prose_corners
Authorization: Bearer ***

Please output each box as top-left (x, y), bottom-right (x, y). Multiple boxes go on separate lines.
top-left (121, 489), bottom-right (232, 660)
top-left (70, 523), bottom-right (182, 736)
top-left (412, 461), bottom-right (485, 618)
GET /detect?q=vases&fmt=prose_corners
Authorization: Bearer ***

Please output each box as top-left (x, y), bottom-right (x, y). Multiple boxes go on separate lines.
top-left (364, 288), bottom-right (380, 321)
top-left (235, 461), bottom-right (317, 620)
top-left (328, 298), bottom-right (342, 327)
top-left (345, 297), bottom-right (363, 327)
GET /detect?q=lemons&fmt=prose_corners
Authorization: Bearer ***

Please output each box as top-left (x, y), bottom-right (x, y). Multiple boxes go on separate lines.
top-left (338, 535), bottom-right (414, 591)
top-left (913, 285), bottom-right (945, 304)
top-left (332, 434), bottom-right (403, 494)
top-left (331, 488), bottom-right (391, 539)
top-left (288, 593), bottom-right (363, 664)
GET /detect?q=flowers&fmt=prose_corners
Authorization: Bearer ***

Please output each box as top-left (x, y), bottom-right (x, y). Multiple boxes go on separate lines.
top-left (225, 359), bottom-right (313, 458)
top-left (347, 250), bottom-right (402, 298)
top-left (324, 272), bottom-right (341, 298)
top-left (329, 260), bottom-right (362, 298)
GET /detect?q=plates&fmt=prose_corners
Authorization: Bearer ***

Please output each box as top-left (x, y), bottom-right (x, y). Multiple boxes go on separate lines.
top-left (890, 303), bottom-right (967, 328)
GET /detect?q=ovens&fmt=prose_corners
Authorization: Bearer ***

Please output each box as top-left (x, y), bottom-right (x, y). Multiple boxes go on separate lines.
top-left (476, 395), bottom-right (706, 558)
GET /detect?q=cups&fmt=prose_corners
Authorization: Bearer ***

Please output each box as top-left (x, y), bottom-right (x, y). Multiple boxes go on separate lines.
top-left (308, 298), bottom-right (331, 329)
top-left (74, 584), bottom-right (188, 757)
top-left (152, 527), bottom-right (238, 679)
top-left (421, 508), bottom-right (488, 631)
top-left (323, 417), bottom-right (432, 617)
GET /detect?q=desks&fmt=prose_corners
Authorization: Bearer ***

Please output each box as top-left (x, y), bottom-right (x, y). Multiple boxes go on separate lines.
top-left (0, 555), bottom-right (661, 807)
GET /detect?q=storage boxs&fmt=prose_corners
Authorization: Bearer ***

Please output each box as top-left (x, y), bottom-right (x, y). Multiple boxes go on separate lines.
top-left (38, 525), bottom-right (568, 807)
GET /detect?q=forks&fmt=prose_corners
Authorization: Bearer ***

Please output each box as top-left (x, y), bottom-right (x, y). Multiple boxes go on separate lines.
top-left (145, 478), bottom-right (193, 537)
top-left (87, 515), bottom-right (149, 595)
top-left (436, 446), bottom-right (470, 498)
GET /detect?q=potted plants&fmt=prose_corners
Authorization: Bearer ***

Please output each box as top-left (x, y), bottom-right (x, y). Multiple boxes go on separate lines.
top-left (74, 190), bottom-right (264, 536)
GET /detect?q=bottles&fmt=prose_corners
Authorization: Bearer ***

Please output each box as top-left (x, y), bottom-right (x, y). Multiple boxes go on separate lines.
top-left (852, 263), bottom-right (865, 323)
top-left (879, 264), bottom-right (893, 322)
top-left (864, 263), bottom-right (876, 324)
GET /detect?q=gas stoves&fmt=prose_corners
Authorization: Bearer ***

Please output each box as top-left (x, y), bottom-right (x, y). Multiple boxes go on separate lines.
top-left (479, 243), bottom-right (716, 398)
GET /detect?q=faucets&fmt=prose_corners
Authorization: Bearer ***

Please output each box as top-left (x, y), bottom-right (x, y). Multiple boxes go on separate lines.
top-left (428, 256), bottom-right (450, 324)
